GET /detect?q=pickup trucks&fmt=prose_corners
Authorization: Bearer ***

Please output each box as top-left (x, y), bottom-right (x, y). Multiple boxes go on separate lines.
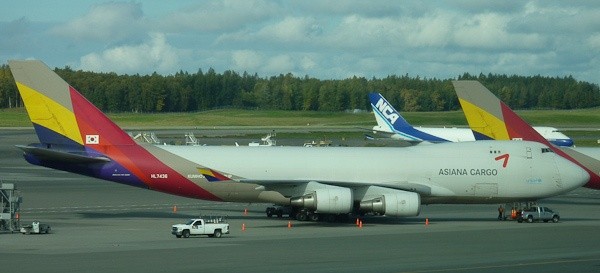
top-left (519, 206), bottom-right (560, 223)
top-left (172, 219), bottom-right (229, 237)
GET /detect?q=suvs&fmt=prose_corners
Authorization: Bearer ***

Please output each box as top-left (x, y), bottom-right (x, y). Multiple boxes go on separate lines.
top-left (267, 200), bottom-right (292, 218)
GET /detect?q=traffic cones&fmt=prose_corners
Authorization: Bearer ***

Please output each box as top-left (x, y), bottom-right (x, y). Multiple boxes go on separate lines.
top-left (16, 211), bottom-right (19, 219)
top-left (244, 207), bottom-right (247, 216)
top-left (355, 218), bottom-right (360, 226)
top-left (359, 221), bottom-right (362, 229)
top-left (426, 218), bottom-right (428, 224)
top-left (288, 221), bottom-right (291, 228)
top-left (174, 205), bottom-right (177, 213)
top-left (242, 224), bottom-right (246, 230)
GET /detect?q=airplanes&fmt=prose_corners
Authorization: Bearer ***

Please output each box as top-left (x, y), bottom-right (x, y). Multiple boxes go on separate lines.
top-left (363, 92), bottom-right (574, 147)
top-left (9, 60), bottom-right (590, 222)
